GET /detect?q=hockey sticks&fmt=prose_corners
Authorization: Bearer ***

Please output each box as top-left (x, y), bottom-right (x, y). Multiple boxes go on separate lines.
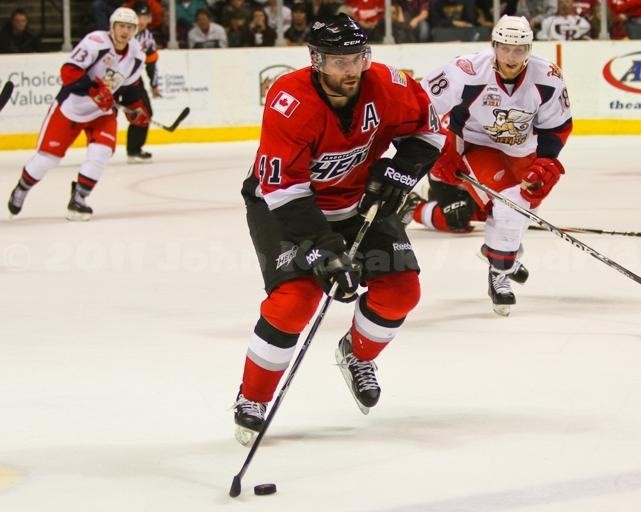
top-left (113, 101), bottom-right (189, 132)
top-left (229, 205), bottom-right (382, 498)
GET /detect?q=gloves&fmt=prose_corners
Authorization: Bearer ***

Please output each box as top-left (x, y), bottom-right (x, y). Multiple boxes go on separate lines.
top-left (304, 233), bottom-right (362, 302)
top-left (89, 77), bottom-right (113, 110)
top-left (125, 100), bottom-right (149, 126)
top-left (521, 159), bottom-right (565, 208)
top-left (432, 131), bottom-right (469, 184)
top-left (358, 137), bottom-right (438, 220)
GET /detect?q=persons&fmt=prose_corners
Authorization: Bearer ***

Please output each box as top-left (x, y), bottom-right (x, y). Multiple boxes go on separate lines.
top-left (122, 1), bottom-right (641, 47)
top-left (400, 103), bottom-right (495, 233)
top-left (422, 14), bottom-right (576, 306)
top-left (9, 6), bottom-right (158, 215)
top-left (232, 10), bottom-right (454, 434)
top-left (113, 1), bottom-right (166, 161)
top-left (0, 10), bottom-right (45, 53)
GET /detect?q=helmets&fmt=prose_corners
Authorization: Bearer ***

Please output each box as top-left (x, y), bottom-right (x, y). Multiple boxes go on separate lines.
top-left (109, 7), bottom-right (138, 43)
top-left (133, 1), bottom-right (152, 14)
top-left (491, 15), bottom-right (533, 64)
top-left (306, 12), bottom-right (372, 74)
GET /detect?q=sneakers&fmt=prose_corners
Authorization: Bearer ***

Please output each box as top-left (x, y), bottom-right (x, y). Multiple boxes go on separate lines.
top-left (128, 147), bottom-right (152, 158)
top-left (234, 383), bottom-right (268, 431)
top-left (488, 272), bottom-right (516, 305)
top-left (338, 326), bottom-right (381, 407)
top-left (481, 245), bottom-right (529, 283)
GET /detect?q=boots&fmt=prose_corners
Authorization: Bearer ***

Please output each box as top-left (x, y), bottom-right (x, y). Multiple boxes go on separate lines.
top-left (9, 178), bottom-right (31, 215)
top-left (68, 182), bottom-right (92, 213)
